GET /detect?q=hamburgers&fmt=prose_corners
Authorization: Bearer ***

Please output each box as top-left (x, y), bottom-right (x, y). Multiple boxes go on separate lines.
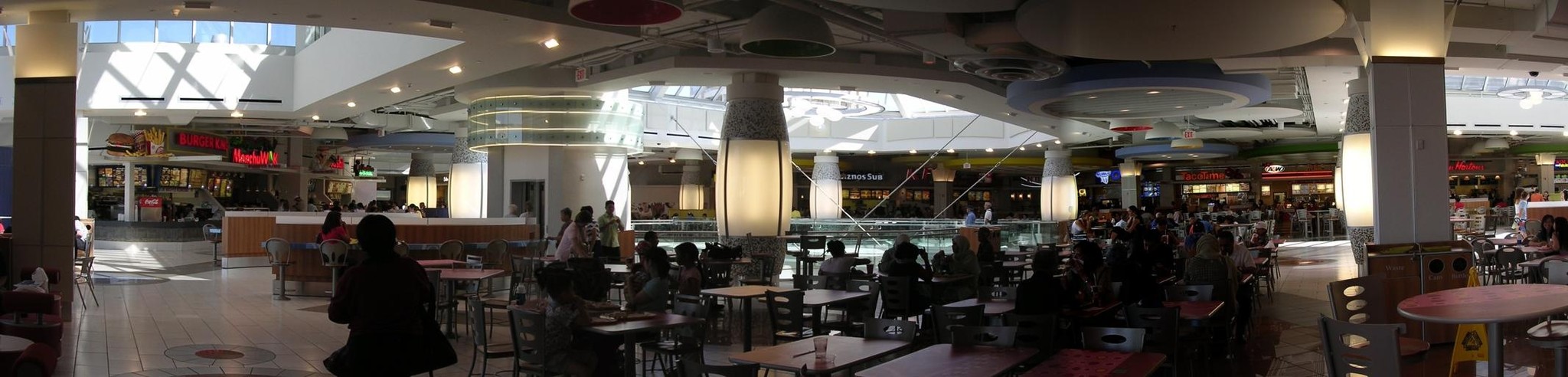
top-left (106, 133), bottom-right (134, 156)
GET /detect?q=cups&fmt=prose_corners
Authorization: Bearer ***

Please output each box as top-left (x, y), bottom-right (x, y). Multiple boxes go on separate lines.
top-left (866, 264), bottom-right (874, 275)
top-left (516, 293), bottom-right (526, 305)
top-left (814, 337), bottom-right (828, 362)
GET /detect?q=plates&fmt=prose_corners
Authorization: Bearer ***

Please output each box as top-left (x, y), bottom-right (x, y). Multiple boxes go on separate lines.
top-left (587, 303), bottom-right (658, 326)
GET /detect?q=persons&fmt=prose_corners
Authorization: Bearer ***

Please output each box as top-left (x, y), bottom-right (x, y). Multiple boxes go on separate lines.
top-left (75, 216), bottom-right (89, 251)
top-left (316, 212), bottom-right (351, 264)
top-left (536, 198), bottom-right (1333, 377)
top-left (290, 196), bottom-right (429, 218)
top-left (323, 215), bottom-right (458, 377)
top-left (505, 204), bottom-right (518, 218)
top-left (1449, 188), bottom-right (1568, 257)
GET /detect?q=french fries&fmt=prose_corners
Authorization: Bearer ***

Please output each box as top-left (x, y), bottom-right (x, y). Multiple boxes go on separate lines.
top-left (143, 126), bottom-right (165, 156)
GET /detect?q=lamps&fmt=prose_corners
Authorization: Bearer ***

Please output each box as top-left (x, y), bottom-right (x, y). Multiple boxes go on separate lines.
top-left (1145, 121), bottom-right (1183, 143)
top-left (1461, 137), bottom-right (1511, 158)
top-left (567, 0), bottom-right (684, 29)
top-left (1109, 118), bottom-right (1154, 132)
top-left (1171, 116), bottom-right (1205, 149)
top-left (738, 5), bottom-right (836, 58)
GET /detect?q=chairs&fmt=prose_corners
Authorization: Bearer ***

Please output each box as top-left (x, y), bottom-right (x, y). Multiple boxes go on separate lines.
top-left (265, 237), bottom-right (295, 301)
top-left (202, 223), bottom-right (224, 267)
top-left (0, 219), bottom-right (100, 377)
top-left (318, 238), bottom-right (348, 302)
